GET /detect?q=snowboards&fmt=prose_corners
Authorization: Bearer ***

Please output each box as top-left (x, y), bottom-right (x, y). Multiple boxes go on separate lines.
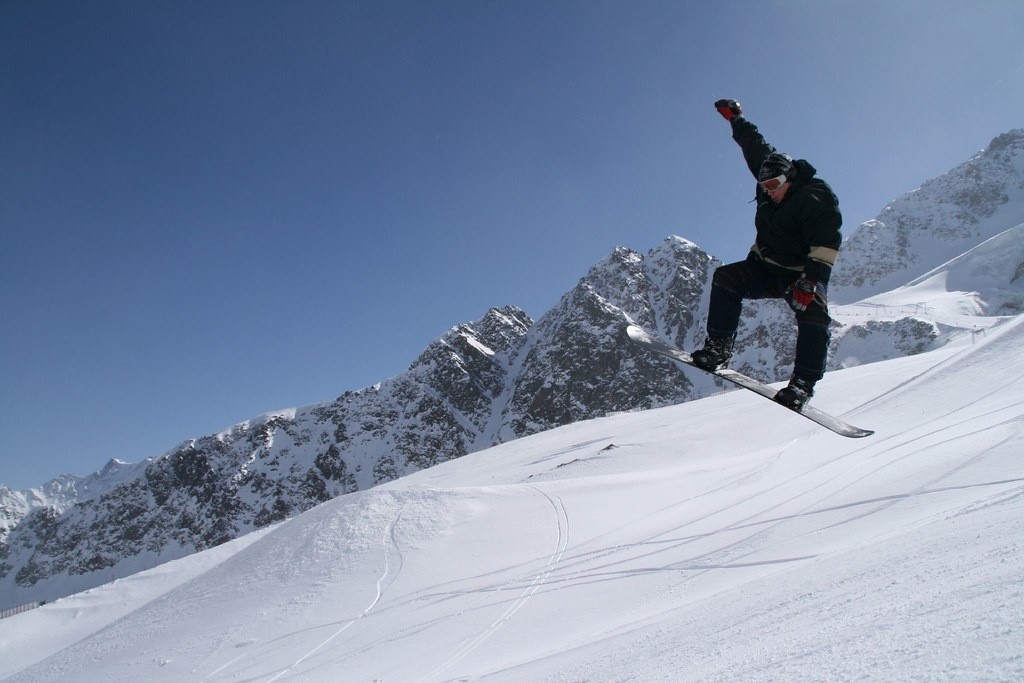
top-left (627, 324), bottom-right (874, 439)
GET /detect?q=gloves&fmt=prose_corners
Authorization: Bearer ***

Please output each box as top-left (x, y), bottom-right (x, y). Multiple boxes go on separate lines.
top-left (715, 98), bottom-right (742, 120)
top-left (792, 272), bottom-right (818, 312)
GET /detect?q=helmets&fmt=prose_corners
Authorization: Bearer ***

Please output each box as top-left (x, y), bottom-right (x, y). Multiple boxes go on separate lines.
top-left (759, 153), bottom-right (795, 184)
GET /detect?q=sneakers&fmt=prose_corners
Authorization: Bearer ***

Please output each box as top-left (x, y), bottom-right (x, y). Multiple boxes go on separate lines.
top-left (778, 371), bottom-right (816, 411)
top-left (692, 335), bottom-right (736, 371)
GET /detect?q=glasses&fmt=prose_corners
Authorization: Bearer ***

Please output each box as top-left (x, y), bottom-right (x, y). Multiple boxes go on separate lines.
top-left (759, 169), bottom-right (795, 192)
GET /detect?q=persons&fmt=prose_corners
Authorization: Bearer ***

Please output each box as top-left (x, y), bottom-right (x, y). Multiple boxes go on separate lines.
top-left (693, 98), bottom-right (842, 410)
